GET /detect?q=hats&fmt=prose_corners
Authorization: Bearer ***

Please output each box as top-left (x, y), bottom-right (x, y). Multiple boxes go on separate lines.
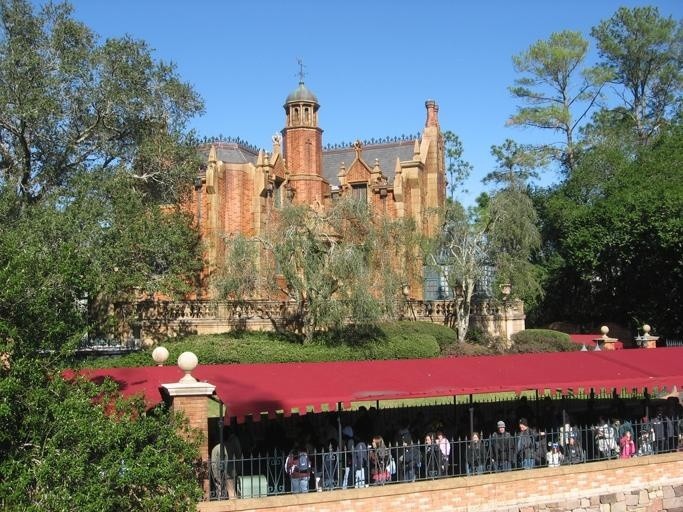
top-left (519, 418), bottom-right (527, 424)
top-left (496, 420), bottom-right (505, 428)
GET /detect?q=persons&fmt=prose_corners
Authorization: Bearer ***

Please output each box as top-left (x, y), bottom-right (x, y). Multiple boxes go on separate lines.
top-left (464, 403), bottom-right (682, 475)
top-left (206, 409), bottom-right (458, 503)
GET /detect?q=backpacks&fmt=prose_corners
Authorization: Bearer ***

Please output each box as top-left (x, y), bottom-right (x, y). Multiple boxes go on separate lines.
top-left (293, 452), bottom-right (311, 472)
